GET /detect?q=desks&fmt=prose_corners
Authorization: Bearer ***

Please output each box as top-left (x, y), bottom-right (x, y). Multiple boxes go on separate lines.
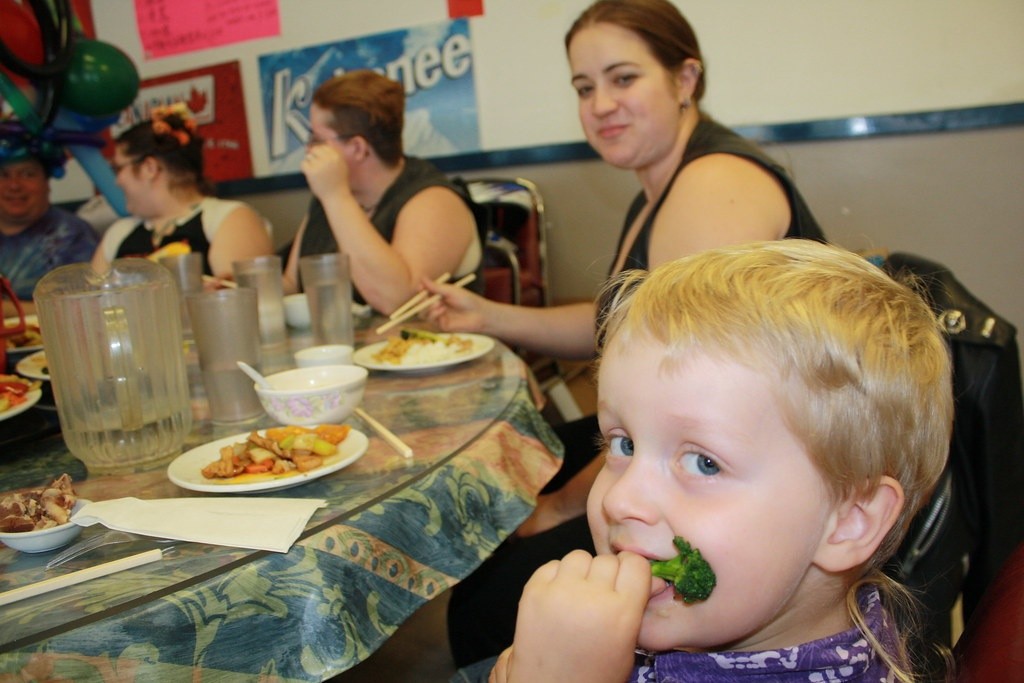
top-left (0, 317), bottom-right (562, 683)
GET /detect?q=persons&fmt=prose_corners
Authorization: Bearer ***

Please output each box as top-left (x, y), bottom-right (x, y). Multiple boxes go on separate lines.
top-left (199, 69), bottom-right (488, 327)
top-left (409, 0), bottom-right (828, 669)
top-left (448, 238), bottom-right (955, 683)
top-left (91, 103), bottom-right (275, 291)
top-left (0, 123), bottom-right (100, 319)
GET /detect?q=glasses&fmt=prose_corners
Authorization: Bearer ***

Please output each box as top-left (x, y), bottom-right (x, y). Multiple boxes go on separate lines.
top-left (306, 125), bottom-right (358, 149)
top-left (110, 146), bottom-right (156, 177)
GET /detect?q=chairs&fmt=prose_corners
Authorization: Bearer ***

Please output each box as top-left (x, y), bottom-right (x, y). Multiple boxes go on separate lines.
top-left (448, 169), bottom-right (559, 356)
top-left (887, 253), bottom-right (1024, 683)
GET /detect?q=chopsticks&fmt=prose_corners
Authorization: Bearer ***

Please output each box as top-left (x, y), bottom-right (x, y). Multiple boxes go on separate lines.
top-left (355, 408), bottom-right (413, 458)
top-left (0, 549), bottom-right (162, 606)
top-left (375, 273), bottom-right (476, 335)
top-left (202, 275), bottom-right (236, 288)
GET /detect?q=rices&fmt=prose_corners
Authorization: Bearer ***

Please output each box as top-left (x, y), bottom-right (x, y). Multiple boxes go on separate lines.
top-left (400, 341), bottom-right (462, 364)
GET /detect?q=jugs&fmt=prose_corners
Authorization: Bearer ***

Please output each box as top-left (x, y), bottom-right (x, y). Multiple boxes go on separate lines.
top-left (33, 259), bottom-right (192, 469)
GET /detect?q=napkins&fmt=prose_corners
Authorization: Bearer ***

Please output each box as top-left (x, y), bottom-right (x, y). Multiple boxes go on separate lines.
top-left (71, 493), bottom-right (330, 554)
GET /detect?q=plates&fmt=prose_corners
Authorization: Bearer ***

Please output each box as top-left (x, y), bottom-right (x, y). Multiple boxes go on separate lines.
top-left (166, 426), bottom-right (368, 496)
top-left (16, 350), bottom-right (50, 380)
top-left (0, 383), bottom-right (42, 421)
top-left (352, 332), bottom-right (495, 374)
top-left (4, 315), bottom-right (43, 353)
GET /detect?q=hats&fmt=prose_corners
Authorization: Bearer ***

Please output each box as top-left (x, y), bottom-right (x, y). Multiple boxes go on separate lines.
top-left (0, 121), bottom-right (41, 164)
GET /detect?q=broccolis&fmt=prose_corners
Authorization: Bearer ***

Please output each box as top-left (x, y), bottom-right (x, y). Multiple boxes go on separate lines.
top-left (650, 536), bottom-right (716, 604)
top-left (399, 327), bottom-right (442, 341)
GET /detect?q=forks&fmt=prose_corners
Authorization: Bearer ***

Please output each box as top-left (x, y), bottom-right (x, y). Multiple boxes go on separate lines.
top-left (44, 530), bottom-right (179, 572)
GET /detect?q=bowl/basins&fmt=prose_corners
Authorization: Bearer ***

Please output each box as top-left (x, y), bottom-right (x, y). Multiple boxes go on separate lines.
top-left (0, 499), bottom-right (91, 554)
top-left (254, 366), bottom-right (369, 428)
top-left (282, 294), bottom-right (311, 329)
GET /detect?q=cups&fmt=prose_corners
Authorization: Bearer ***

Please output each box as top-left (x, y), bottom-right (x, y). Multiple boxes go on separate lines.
top-left (293, 344), bottom-right (352, 369)
top-left (231, 255), bottom-right (287, 349)
top-left (158, 252), bottom-right (201, 334)
top-left (299, 253), bottom-right (354, 346)
